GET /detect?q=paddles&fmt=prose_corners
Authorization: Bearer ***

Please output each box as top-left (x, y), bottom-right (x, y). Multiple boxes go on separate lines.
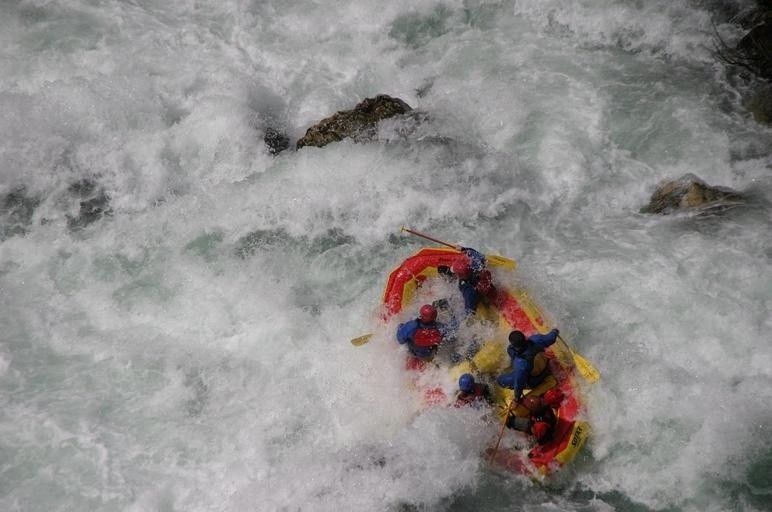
top-left (400, 225), bottom-right (517, 272)
top-left (520, 292), bottom-right (600, 384)
top-left (351, 333), bottom-right (375, 347)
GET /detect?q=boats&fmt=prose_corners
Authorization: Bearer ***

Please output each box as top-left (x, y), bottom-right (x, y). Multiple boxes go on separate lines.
top-left (380, 245), bottom-right (590, 481)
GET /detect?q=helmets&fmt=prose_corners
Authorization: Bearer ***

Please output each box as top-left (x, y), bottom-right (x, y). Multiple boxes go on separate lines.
top-left (525, 395), bottom-right (543, 411)
top-left (453, 261), bottom-right (470, 274)
top-left (459, 374), bottom-right (474, 391)
top-left (420, 304), bottom-right (437, 324)
top-left (509, 331), bottom-right (525, 347)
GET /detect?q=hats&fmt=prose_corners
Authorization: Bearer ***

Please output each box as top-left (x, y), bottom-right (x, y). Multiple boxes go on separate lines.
top-left (450, 265), bottom-right (455, 273)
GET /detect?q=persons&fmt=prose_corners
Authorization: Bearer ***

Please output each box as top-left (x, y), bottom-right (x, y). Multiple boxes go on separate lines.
top-left (508, 389), bottom-right (562, 457)
top-left (496, 329), bottom-right (559, 407)
top-left (455, 374), bottom-right (489, 407)
top-left (397, 305), bottom-right (448, 370)
top-left (450, 246), bottom-right (496, 316)
